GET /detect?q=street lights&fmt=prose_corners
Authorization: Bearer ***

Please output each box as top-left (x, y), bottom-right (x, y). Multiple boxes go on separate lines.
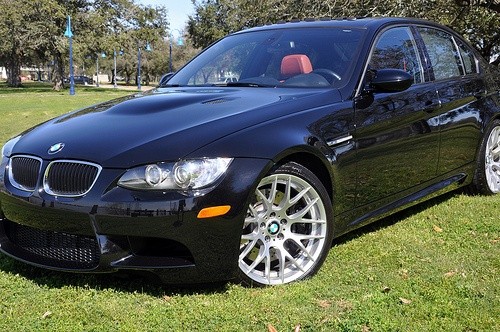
top-left (169, 29), bottom-right (183, 74)
top-left (64, 15), bottom-right (76, 95)
top-left (136, 39), bottom-right (152, 91)
top-left (96, 49), bottom-right (106, 87)
top-left (113, 46), bottom-right (123, 87)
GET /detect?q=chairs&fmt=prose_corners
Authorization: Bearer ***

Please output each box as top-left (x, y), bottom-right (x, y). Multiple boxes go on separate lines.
top-left (274, 54), bottom-right (313, 88)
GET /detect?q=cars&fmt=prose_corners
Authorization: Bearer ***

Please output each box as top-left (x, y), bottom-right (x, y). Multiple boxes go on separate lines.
top-left (64, 75), bottom-right (92, 85)
top-left (0, 16), bottom-right (500, 287)
